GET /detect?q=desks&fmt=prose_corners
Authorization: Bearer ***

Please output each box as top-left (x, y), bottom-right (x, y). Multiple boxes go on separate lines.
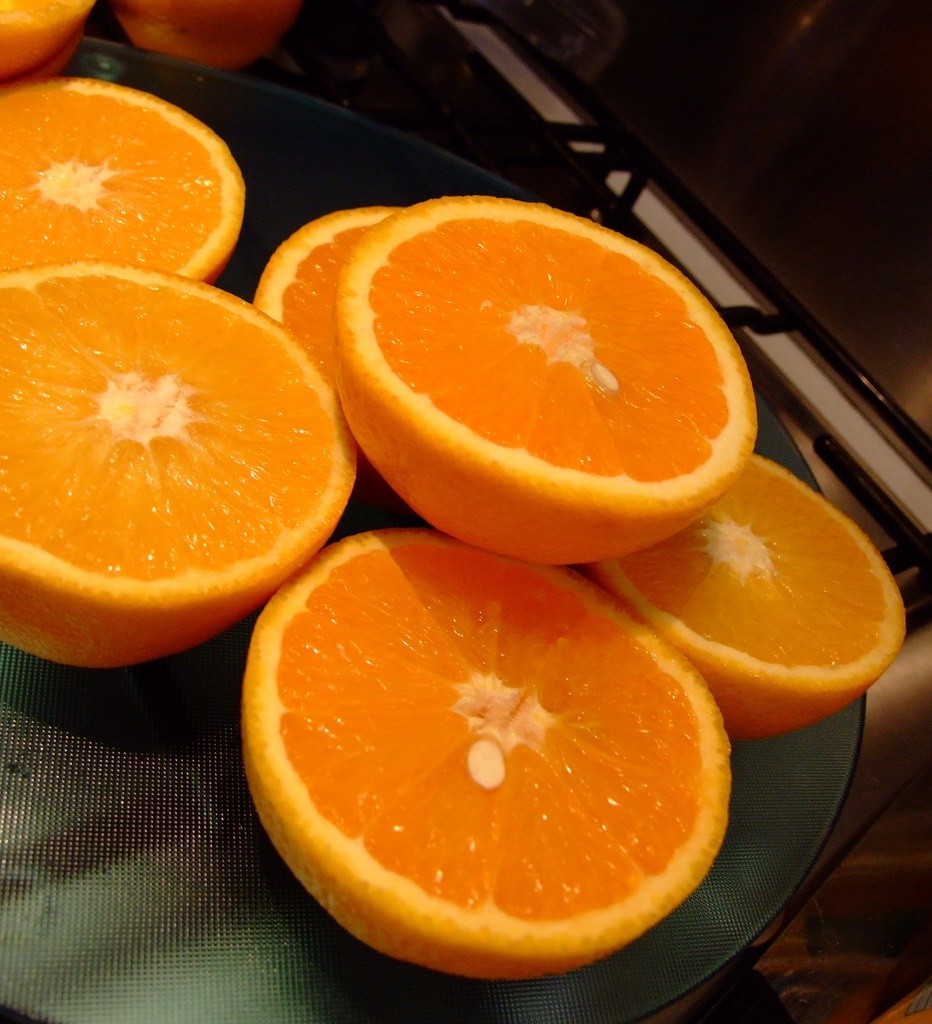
top-left (1, 30), bottom-right (870, 1024)
